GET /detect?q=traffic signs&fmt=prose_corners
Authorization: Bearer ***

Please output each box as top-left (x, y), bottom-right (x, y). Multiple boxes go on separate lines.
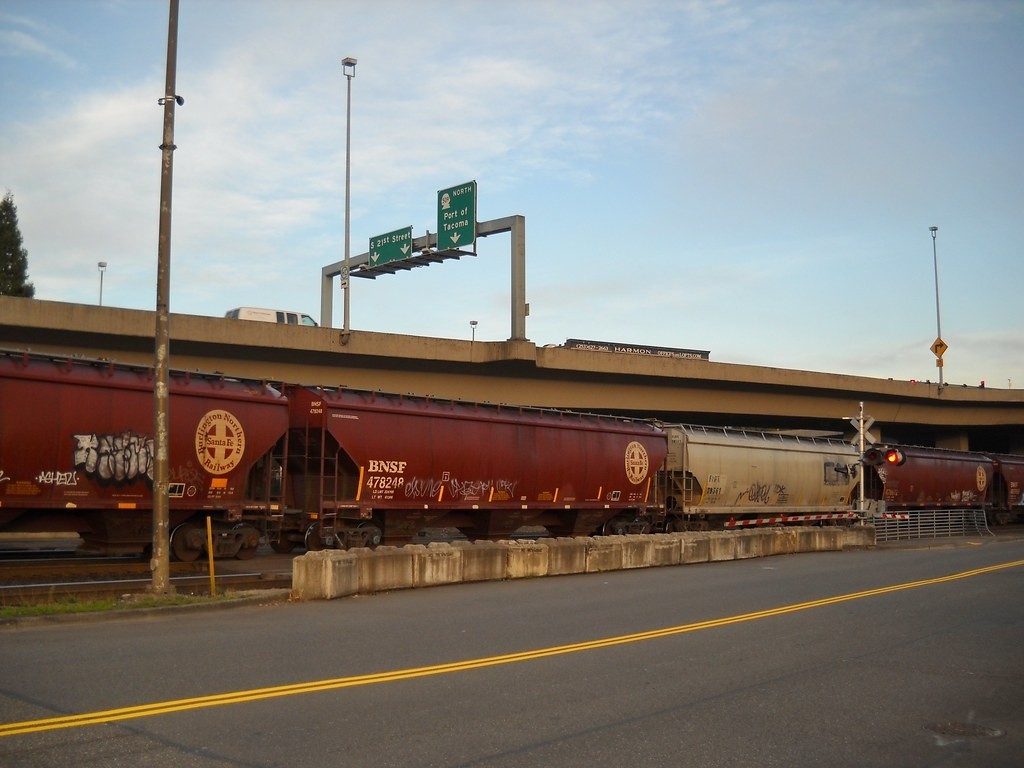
top-left (369, 225), bottom-right (413, 269)
top-left (436, 180), bottom-right (477, 254)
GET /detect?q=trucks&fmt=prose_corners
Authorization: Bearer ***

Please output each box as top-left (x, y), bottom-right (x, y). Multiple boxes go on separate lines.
top-left (543, 338), bottom-right (709, 361)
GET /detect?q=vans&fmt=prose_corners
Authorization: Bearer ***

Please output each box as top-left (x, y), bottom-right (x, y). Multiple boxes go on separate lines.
top-left (223, 307), bottom-right (320, 327)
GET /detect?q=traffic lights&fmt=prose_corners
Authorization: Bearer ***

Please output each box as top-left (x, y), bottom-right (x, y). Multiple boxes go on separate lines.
top-left (886, 449), bottom-right (907, 468)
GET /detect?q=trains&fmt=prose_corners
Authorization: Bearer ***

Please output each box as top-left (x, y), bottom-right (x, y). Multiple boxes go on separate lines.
top-left (0, 352), bottom-right (1023, 561)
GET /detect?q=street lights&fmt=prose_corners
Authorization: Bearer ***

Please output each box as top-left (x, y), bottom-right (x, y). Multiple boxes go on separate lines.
top-left (97, 261), bottom-right (108, 306)
top-left (930, 225), bottom-right (944, 383)
top-left (340, 56), bottom-right (358, 335)
